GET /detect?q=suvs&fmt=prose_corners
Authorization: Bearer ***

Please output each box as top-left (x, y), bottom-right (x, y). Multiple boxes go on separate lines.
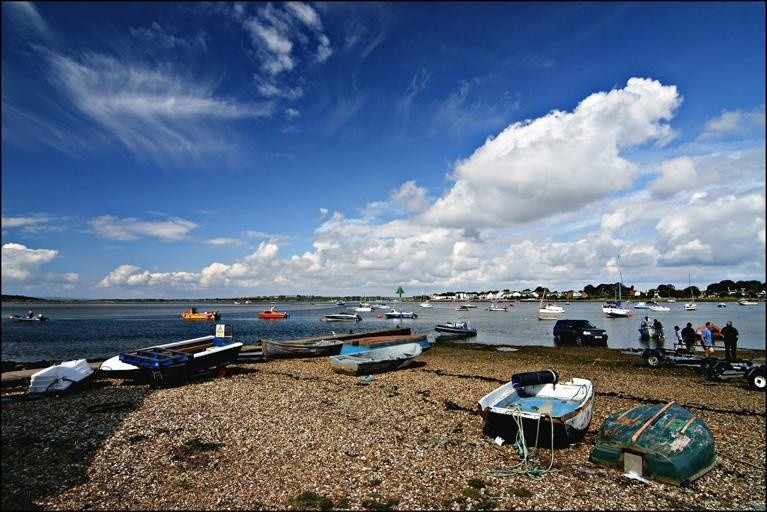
top-left (552, 319), bottom-right (607, 348)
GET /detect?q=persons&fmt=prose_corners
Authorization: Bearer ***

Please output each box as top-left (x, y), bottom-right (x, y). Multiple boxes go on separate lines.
top-left (700, 321), bottom-right (713, 358)
top-left (639, 315), bottom-right (652, 327)
top-left (681, 322), bottom-right (697, 351)
top-left (720, 320), bottom-right (739, 359)
top-left (652, 319), bottom-right (664, 339)
top-left (674, 326), bottom-right (682, 344)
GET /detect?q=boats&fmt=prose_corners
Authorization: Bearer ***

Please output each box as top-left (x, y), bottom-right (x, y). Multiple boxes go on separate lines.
top-left (97, 334), bottom-right (243, 384)
top-left (26, 356), bottom-right (94, 398)
top-left (9, 313), bottom-right (49, 324)
top-left (589, 398), bottom-right (719, 487)
top-left (476, 367), bottom-right (596, 451)
top-left (600, 298), bottom-right (759, 317)
top-left (565, 301), bottom-right (570, 305)
top-left (694, 319), bottom-right (725, 340)
top-left (637, 326), bottom-right (658, 339)
top-left (234, 298), bottom-right (514, 378)
top-left (536, 286), bottom-right (564, 320)
top-left (179, 307), bottom-right (222, 320)
